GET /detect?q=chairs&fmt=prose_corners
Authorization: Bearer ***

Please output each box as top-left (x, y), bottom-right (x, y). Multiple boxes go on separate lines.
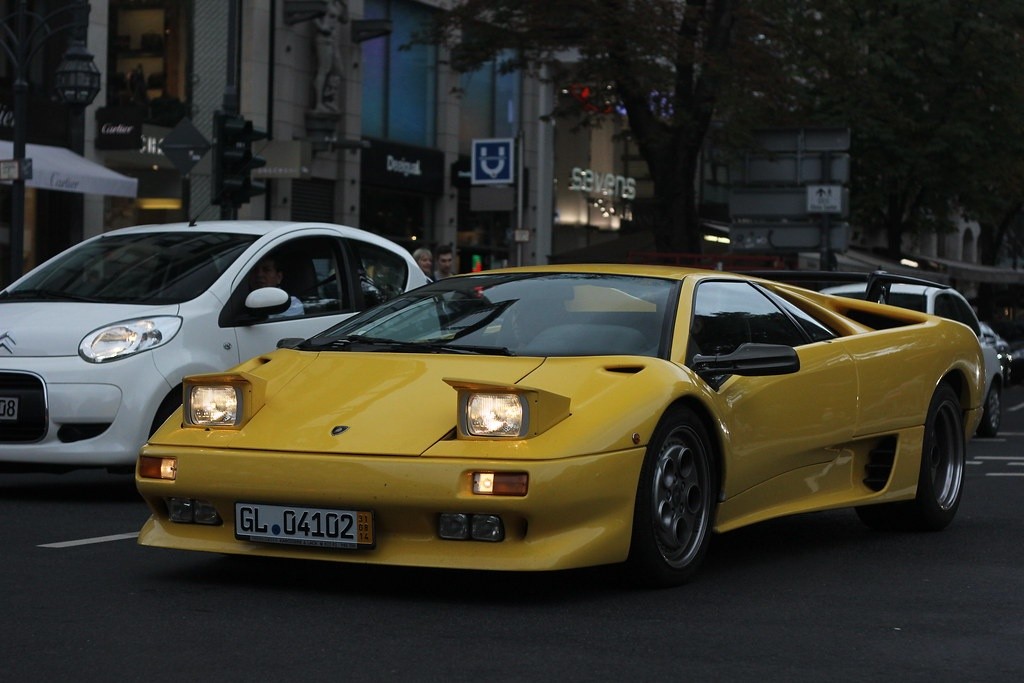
top-left (283, 266), bottom-right (313, 315)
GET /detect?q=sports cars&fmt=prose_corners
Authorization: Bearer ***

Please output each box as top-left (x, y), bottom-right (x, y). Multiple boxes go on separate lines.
top-left (135, 264), bottom-right (986, 588)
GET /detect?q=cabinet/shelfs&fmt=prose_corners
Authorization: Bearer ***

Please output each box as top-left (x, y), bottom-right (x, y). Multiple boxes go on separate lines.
top-left (110, 2), bottom-right (180, 97)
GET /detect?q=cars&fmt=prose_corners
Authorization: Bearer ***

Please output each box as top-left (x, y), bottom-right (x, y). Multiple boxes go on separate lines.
top-left (0, 220), bottom-right (446, 473)
top-left (818, 276), bottom-right (1024, 437)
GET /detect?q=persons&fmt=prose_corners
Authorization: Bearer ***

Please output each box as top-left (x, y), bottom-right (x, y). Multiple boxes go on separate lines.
top-left (434, 245), bottom-right (453, 282)
top-left (249, 251), bottom-right (306, 321)
top-left (313, 0), bottom-right (348, 112)
top-left (493, 292), bottom-right (568, 351)
top-left (647, 286), bottom-right (704, 364)
top-left (413, 248), bottom-right (434, 278)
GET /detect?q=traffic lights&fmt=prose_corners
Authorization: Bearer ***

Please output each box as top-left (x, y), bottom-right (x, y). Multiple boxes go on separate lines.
top-left (219, 119), bottom-right (268, 201)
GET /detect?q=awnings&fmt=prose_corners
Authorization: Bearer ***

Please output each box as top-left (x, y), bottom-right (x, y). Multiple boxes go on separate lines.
top-left (0, 141), bottom-right (138, 200)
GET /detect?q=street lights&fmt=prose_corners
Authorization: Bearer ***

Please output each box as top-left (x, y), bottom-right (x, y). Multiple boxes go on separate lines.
top-left (0, 0), bottom-right (102, 282)
